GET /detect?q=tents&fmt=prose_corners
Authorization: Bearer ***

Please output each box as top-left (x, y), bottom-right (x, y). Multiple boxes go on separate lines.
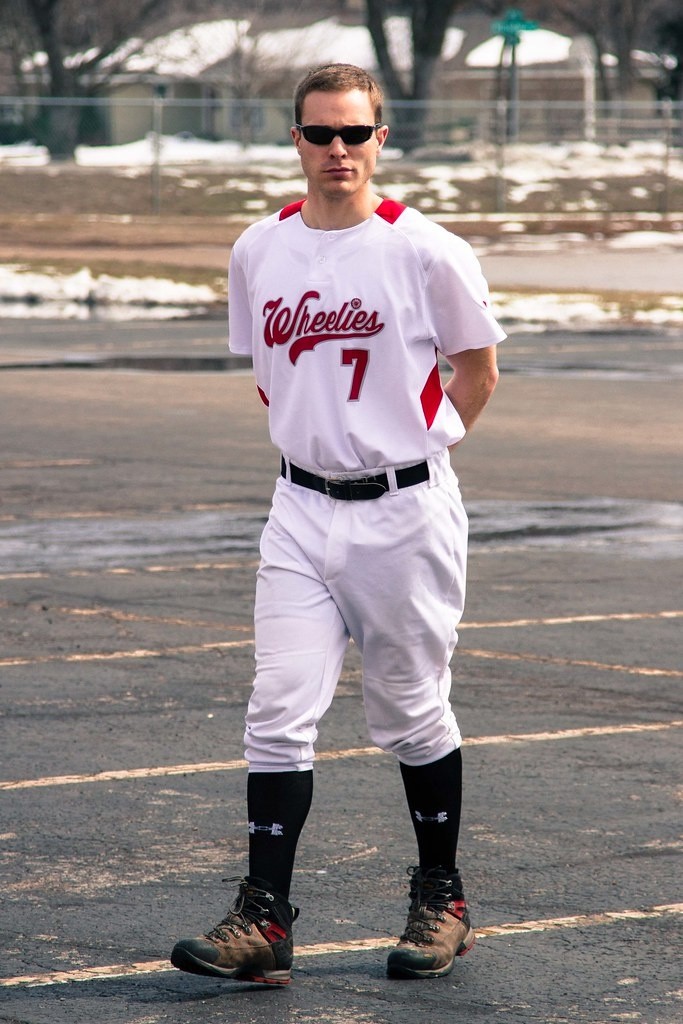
top-left (16, 13), bottom-right (677, 143)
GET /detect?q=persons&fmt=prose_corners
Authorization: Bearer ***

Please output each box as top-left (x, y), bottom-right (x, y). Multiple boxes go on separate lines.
top-left (171, 62), bottom-right (507, 985)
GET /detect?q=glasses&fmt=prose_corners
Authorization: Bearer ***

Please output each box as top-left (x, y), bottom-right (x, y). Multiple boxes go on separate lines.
top-left (295, 123), bottom-right (382, 146)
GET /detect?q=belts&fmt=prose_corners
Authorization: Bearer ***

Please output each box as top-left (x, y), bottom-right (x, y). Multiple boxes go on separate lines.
top-left (281, 455), bottom-right (429, 500)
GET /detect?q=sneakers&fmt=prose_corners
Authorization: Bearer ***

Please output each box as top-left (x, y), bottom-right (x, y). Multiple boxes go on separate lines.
top-left (386, 867), bottom-right (477, 978)
top-left (171, 876), bottom-right (300, 986)
